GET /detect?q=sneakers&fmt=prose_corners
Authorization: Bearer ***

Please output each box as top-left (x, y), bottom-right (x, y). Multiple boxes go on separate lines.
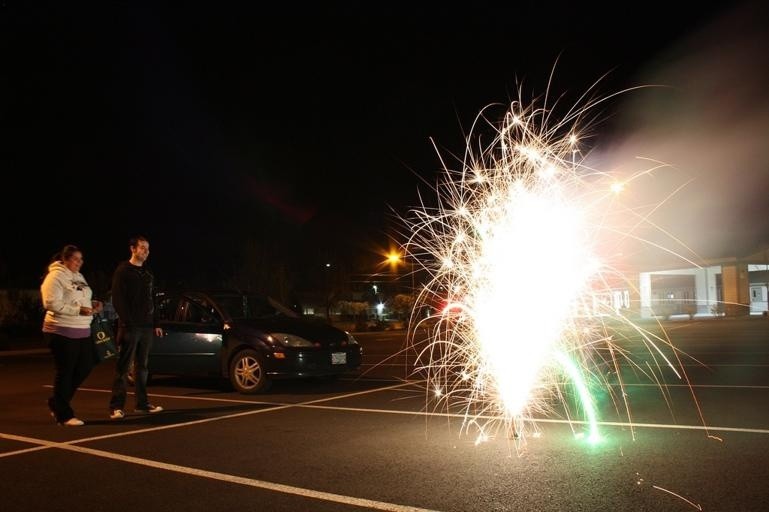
top-left (134, 403), bottom-right (164, 414)
top-left (63, 418), bottom-right (85, 426)
top-left (46, 397), bottom-right (60, 427)
top-left (109, 407), bottom-right (125, 419)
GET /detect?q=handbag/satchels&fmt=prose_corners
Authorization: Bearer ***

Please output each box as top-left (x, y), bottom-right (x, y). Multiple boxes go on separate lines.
top-left (89, 312), bottom-right (118, 362)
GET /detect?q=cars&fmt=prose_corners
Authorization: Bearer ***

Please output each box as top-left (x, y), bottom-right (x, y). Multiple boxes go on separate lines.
top-left (115, 290), bottom-right (366, 395)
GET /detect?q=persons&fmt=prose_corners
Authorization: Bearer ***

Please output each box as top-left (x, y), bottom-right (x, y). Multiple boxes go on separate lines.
top-left (39, 245), bottom-right (102, 428)
top-left (109, 238), bottom-right (163, 419)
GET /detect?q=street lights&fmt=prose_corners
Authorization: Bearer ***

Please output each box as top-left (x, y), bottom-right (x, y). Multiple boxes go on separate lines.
top-left (386, 251), bottom-right (415, 295)
top-left (325, 263), bottom-right (334, 322)
